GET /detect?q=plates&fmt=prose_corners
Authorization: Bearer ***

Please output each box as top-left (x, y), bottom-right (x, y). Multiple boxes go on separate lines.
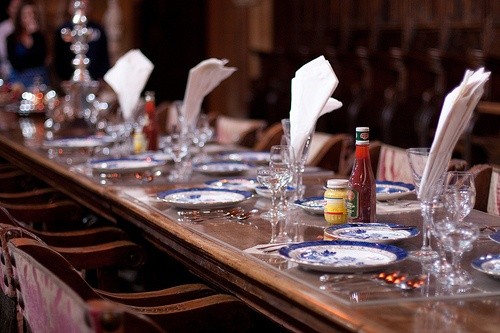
top-left (277, 241), bottom-right (408, 274)
top-left (373, 179), bottom-right (416, 200)
top-left (215, 147), bottom-right (282, 165)
top-left (255, 183), bottom-right (295, 198)
top-left (88, 157), bottom-right (167, 173)
top-left (154, 187), bottom-right (254, 209)
top-left (206, 176), bottom-right (257, 192)
top-left (42, 136), bottom-right (111, 147)
top-left (132, 150), bottom-right (178, 162)
top-left (471, 253), bottom-right (500, 276)
top-left (195, 162), bottom-right (251, 175)
top-left (295, 196), bottom-right (328, 214)
top-left (487, 230), bottom-right (500, 244)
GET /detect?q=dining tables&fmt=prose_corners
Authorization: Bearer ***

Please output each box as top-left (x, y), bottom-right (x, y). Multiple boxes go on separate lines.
top-left (0, 110), bottom-right (500, 333)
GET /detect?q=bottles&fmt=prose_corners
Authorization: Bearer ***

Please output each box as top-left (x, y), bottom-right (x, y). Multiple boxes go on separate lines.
top-left (347, 125), bottom-right (378, 223)
top-left (323, 220), bottom-right (420, 244)
top-left (322, 179), bottom-right (349, 223)
top-left (140, 92), bottom-right (161, 152)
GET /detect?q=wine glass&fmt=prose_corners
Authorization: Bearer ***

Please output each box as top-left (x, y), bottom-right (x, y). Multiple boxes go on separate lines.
top-left (92, 67), bottom-right (315, 250)
top-left (406, 145), bottom-right (480, 286)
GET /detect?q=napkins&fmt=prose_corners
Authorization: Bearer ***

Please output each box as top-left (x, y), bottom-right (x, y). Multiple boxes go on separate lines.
top-left (418, 67), bottom-right (491, 199)
top-left (289, 55), bottom-right (342, 161)
top-left (182, 58), bottom-right (236, 124)
top-left (104, 49), bottom-right (154, 118)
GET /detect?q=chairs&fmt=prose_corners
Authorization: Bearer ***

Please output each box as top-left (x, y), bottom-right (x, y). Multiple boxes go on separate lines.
top-left (0, 77), bottom-right (500, 333)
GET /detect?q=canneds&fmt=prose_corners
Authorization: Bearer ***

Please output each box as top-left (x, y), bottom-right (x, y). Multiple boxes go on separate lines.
top-left (323, 179), bottom-right (351, 225)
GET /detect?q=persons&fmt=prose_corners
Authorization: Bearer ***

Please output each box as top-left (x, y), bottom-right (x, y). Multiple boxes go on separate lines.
top-left (0, 0), bottom-right (112, 98)
